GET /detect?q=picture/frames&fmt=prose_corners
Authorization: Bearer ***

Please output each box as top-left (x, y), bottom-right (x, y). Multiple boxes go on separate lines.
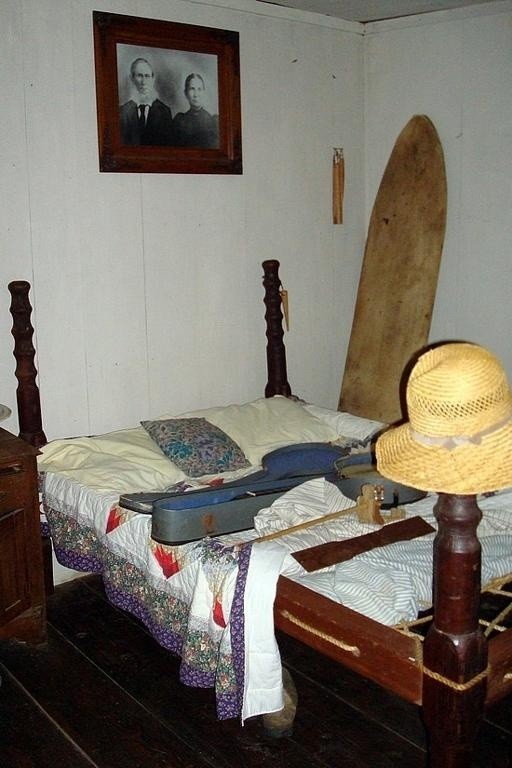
top-left (91, 10), bottom-right (244, 176)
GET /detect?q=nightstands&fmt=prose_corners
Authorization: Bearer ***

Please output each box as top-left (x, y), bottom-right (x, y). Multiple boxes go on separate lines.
top-left (0, 426), bottom-right (48, 647)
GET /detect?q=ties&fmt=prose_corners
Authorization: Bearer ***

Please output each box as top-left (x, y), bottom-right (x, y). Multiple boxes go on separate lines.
top-left (139, 103), bottom-right (146, 126)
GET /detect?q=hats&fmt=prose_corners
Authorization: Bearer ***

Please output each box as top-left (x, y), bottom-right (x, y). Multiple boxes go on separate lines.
top-left (375, 342), bottom-right (512, 496)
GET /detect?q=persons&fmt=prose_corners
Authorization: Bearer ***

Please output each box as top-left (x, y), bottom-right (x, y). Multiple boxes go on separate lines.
top-left (118, 57), bottom-right (173, 146)
top-left (173, 72), bottom-right (219, 149)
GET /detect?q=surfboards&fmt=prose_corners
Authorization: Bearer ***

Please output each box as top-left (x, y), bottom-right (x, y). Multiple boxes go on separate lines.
top-left (338, 115), bottom-right (446, 425)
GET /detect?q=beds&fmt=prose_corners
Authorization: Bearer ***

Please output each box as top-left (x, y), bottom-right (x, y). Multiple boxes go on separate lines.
top-left (38, 393), bottom-right (512, 766)
